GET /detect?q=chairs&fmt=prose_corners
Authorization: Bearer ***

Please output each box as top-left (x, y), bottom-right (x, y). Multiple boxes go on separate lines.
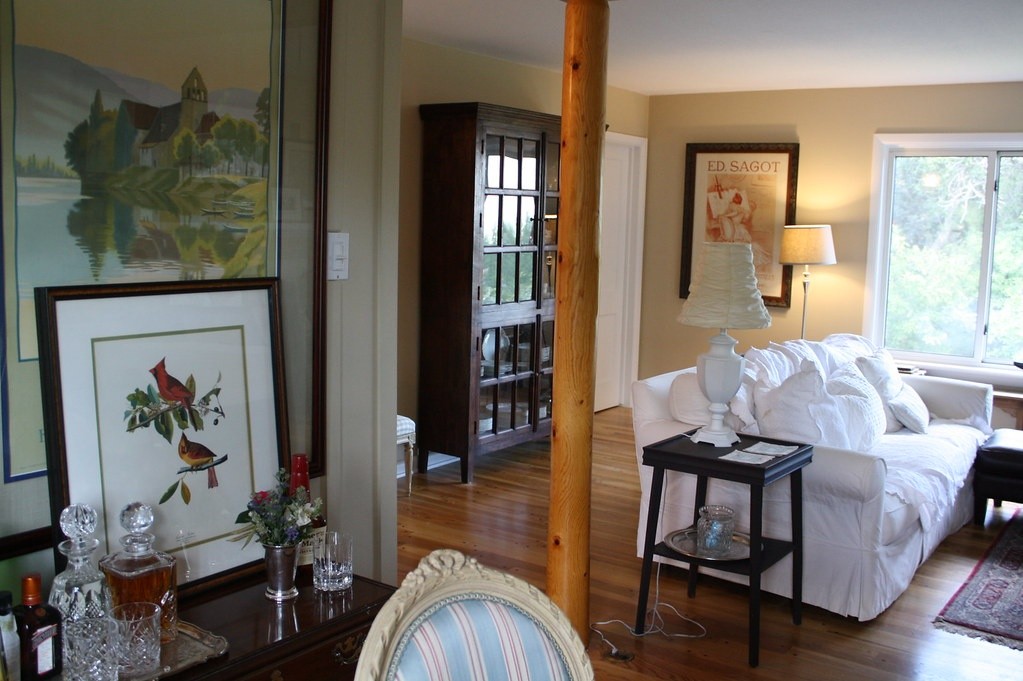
top-left (397, 414), bottom-right (416, 496)
top-left (354, 549), bottom-right (596, 681)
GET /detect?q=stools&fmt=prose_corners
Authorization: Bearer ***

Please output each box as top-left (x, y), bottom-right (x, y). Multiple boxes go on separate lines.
top-left (972, 429), bottom-right (1023, 526)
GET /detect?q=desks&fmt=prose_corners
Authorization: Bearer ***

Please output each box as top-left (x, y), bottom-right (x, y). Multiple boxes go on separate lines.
top-left (634, 427), bottom-right (815, 668)
top-left (134, 563), bottom-right (399, 681)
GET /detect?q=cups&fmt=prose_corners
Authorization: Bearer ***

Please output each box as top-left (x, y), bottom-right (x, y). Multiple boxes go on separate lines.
top-left (108, 602), bottom-right (161, 678)
top-left (312, 531), bottom-right (353, 591)
top-left (61, 618), bottom-right (118, 681)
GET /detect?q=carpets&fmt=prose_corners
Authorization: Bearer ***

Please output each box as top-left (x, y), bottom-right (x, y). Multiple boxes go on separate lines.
top-left (932, 508), bottom-right (1022, 652)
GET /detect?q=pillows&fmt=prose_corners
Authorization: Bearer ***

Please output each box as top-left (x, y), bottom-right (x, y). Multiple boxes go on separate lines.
top-left (672, 335), bottom-right (931, 452)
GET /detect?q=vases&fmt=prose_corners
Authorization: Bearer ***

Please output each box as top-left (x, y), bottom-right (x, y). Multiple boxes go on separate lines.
top-left (261, 543), bottom-right (302, 601)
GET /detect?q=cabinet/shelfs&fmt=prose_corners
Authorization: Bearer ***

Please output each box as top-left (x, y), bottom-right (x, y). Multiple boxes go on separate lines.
top-left (417, 101), bottom-right (561, 485)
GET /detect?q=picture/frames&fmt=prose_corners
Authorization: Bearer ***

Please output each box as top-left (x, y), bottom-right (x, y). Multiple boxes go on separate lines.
top-left (0, 0), bottom-right (288, 484)
top-left (679, 142), bottom-right (800, 308)
top-left (33, 276), bottom-right (292, 594)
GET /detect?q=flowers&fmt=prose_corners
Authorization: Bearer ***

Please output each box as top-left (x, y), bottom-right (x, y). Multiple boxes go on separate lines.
top-left (225, 468), bottom-right (323, 551)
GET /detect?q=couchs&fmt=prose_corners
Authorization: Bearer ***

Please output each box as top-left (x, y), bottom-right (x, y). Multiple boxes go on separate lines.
top-left (630, 340), bottom-right (995, 625)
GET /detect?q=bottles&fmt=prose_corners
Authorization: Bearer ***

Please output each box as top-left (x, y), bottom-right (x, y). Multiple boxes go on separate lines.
top-left (697, 505), bottom-right (734, 556)
top-left (99, 502), bottom-right (177, 645)
top-left (0, 574), bottom-right (62, 681)
top-left (288, 454), bottom-right (326, 586)
top-left (48, 504), bottom-right (113, 622)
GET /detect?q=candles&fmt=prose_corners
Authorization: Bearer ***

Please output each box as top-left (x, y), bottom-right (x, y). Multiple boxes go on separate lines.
top-left (291, 454), bottom-right (311, 500)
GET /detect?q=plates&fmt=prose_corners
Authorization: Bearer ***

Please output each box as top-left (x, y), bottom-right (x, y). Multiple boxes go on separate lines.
top-left (480, 328), bottom-right (550, 376)
top-left (479, 401), bottom-right (548, 435)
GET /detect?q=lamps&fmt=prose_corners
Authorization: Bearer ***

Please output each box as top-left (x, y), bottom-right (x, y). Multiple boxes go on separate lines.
top-left (676, 240), bottom-right (773, 447)
top-left (779, 224), bottom-right (836, 340)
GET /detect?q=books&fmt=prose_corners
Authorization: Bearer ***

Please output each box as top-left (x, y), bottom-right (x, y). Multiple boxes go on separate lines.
top-left (897, 365), bottom-right (920, 374)
